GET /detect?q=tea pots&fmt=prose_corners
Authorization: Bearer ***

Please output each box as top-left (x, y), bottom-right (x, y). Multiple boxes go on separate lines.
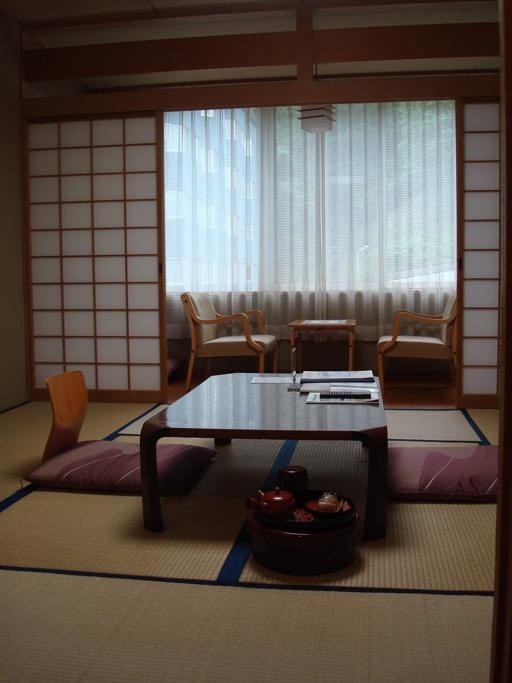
top-left (248, 486), bottom-right (296, 518)
top-left (316, 489), bottom-right (344, 512)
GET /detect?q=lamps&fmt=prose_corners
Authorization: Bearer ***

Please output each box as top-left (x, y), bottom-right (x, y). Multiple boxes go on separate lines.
top-left (297, 104), bottom-right (337, 133)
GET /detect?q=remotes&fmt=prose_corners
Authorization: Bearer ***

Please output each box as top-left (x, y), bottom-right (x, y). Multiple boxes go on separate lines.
top-left (320, 389), bottom-right (371, 399)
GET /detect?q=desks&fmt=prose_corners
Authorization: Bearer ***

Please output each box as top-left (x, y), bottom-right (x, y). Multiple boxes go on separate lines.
top-left (140, 373), bottom-right (388, 541)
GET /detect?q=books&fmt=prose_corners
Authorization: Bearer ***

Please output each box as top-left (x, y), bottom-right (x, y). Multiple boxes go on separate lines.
top-left (300, 370), bottom-right (379, 404)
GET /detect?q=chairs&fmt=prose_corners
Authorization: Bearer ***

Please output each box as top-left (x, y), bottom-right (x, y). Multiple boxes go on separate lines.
top-left (377, 292), bottom-right (457, 395)
top-left (181, 291), bottom-right (278, 393)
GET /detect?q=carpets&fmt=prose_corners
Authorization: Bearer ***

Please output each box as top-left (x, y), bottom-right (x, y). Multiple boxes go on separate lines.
top-left (0, 403), bottom-right (496, 596)
